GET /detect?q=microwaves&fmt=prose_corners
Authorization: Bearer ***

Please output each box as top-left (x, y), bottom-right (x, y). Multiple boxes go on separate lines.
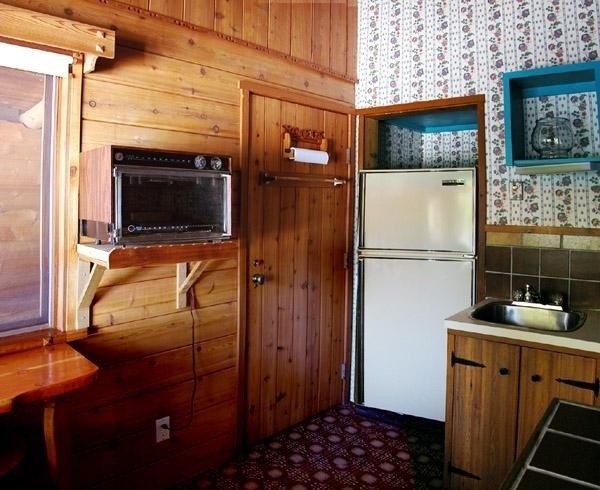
top-left (78, 146), bottom-right (233, 250)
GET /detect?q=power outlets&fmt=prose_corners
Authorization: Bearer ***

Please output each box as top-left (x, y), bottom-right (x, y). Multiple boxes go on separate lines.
top-left (156, 416), bottom-right (170, 444)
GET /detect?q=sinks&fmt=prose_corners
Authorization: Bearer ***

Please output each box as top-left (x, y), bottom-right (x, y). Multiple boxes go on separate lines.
top-left (468, 300), bottom-right (585, 332)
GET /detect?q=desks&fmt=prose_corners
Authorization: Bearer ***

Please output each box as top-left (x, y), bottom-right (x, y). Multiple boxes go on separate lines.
top-left (0, 343), bottom-right (100, 490)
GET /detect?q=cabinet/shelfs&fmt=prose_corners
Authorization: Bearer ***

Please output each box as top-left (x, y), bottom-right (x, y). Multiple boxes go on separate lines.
top-left (502, 60), bottom-right (600, 167)
top-left (450, 336), bottom-right (596, 490)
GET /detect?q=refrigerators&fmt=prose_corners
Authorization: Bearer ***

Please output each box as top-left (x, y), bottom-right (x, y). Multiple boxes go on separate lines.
top-left (359, 171), bottom-right (475, 421)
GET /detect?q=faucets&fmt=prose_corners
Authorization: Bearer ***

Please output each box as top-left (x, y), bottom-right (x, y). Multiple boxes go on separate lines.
top-left (524, 284), bottom-right (538, 302)
